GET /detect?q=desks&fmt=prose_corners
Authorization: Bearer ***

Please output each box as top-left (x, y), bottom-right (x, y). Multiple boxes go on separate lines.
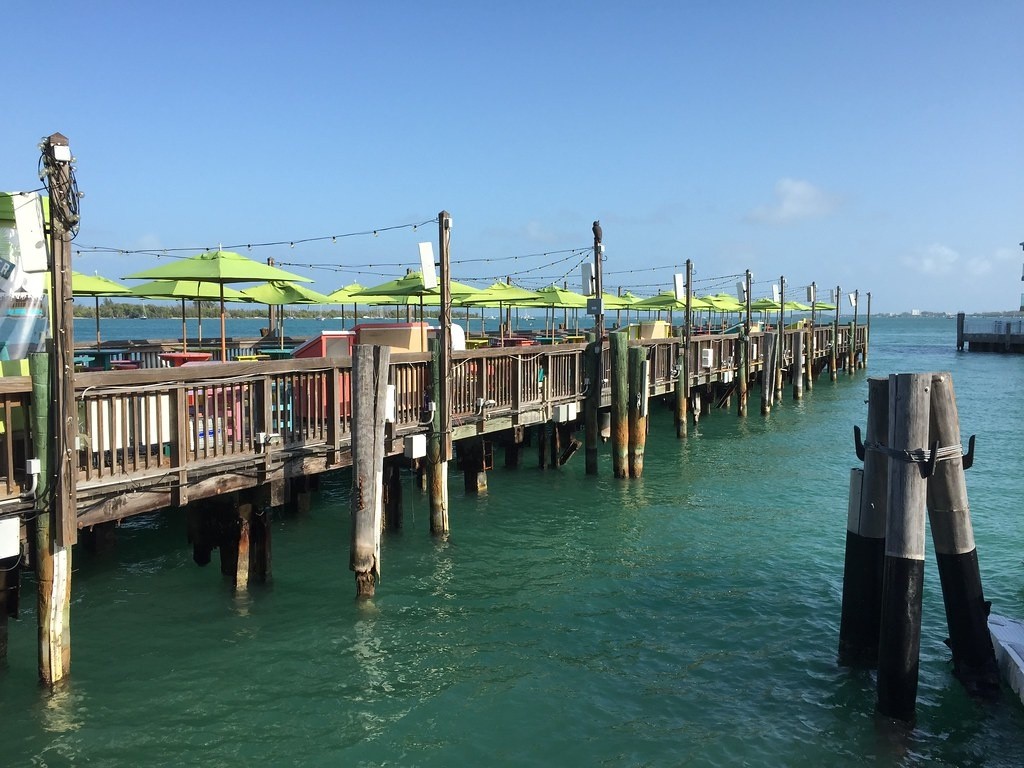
top-left (72, 343), bottom-right (298, 440)
top-left (427, 325), bottom-right (598, 394)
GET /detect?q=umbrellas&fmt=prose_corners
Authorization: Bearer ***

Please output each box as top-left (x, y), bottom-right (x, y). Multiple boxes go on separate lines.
top-left (129, 280), bottom-right (255, 354)
top-left (329, 268), bottom-right (492, 351)
top-left (801, 300), bottom-right (836, 327)
top-left (461, 279), bottom-right (812, 345)
top-left (241, 281), bottom-right (337, 350)
top-left (44, 271), bottom-right (132, 352)
top-left (120, 243), bottom-right (314, 363)
